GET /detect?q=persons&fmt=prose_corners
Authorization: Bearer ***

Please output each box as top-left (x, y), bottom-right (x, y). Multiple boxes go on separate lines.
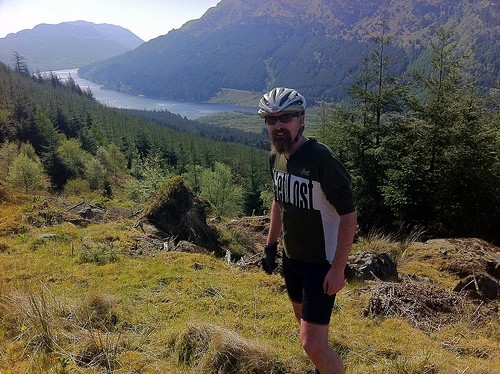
top-left (251, 87), bottom-right (362, 374)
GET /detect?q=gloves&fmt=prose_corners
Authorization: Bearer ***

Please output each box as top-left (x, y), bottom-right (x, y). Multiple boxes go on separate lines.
top-left (260, 244), bottom-right (279, 276)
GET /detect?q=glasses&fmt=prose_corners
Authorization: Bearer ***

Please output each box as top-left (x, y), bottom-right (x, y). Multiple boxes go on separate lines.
top-left (264, 113), bottom-right (300, 125)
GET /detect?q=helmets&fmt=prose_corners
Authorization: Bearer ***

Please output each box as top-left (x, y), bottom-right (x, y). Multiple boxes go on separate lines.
top-left (257, 87), bottom-right (308, 119)
